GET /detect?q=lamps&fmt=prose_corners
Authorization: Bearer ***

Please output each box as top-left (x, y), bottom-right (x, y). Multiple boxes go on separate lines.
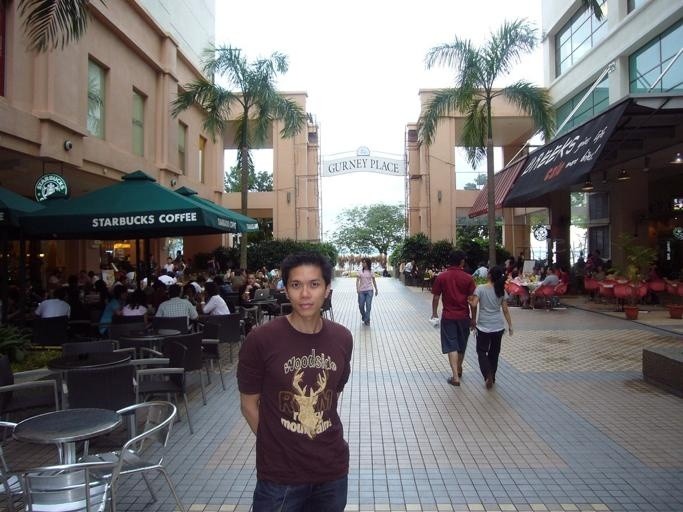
top-left (581, 141), bottom-right (683, 192)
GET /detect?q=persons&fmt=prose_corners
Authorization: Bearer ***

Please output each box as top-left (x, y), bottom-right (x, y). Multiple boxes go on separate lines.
top-left (472, 265), bottom-right (513, 388)
top-left (429, 250), bottom-right (476, 386)
top-left (5, 249), bottom-right (284, 339)
top-left (402, 249), bottom-right (603, 309)
top-left (235, 252), bottom-right (352, 511)
top-left (355, 257), bottom-right (377, 325)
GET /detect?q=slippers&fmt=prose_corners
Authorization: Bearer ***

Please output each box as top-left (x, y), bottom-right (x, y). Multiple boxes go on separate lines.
top-left (458, 368), bottom-right (462, 376)
top-left (447, 377), bottom-right (460, 386)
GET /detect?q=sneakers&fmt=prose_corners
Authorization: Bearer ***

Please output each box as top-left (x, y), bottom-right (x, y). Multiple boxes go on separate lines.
top-left (362, 317), bottom-right (365, 321)
top-left (486, 377), bottom-right (493, 390)
top-left (364, 320), bottom-right (370, 326)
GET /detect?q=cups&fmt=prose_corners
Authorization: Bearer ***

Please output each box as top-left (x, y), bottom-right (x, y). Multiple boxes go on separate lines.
top-left (244, 291), bottom-right (250, 300)
top-left (200, 302), bottom-right (205, 308)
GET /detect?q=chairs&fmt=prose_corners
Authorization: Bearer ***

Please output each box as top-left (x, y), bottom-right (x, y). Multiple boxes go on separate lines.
top-left (503, 261), bottom-right (683, 310)
top-left (320, 289), bottom-right (335, 321)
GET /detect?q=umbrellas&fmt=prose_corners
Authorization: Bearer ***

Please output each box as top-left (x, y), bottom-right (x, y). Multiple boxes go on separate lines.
top-left (0, 170), bottom-right (259, 308)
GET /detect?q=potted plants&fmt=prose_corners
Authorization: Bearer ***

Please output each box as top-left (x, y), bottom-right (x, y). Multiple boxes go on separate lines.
top-left (612, 231), bottom-right (658, 320)
top-left (665, 265), bottom-right (683, 318)
